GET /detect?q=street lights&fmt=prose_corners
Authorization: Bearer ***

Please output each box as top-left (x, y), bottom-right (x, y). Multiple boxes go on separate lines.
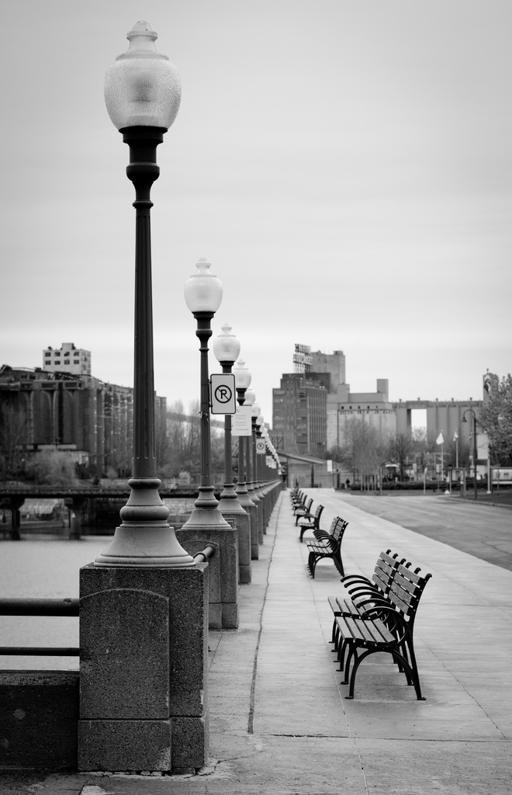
top-left (229, 359), bottom-right (285, 505)
top-left (82, 18), bottom-right (187, 543)
top-left (461, 408), bottom-right (478, 499)
top-left (214, 323), bottom-right (249, 518)
top-left (172, 258), bottom-right (234, 530)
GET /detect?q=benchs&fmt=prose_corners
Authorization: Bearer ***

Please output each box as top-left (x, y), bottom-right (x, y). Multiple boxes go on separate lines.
top-left (289, 484), bottom-right (432, 701)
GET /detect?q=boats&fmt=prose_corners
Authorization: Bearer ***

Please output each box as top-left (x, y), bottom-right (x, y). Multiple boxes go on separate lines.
top-left (0, 498), bottom-right (74, 539)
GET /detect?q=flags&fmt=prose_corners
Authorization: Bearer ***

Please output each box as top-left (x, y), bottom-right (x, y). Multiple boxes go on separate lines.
top-left (452, 431), bottom-right (458, 443)
top-left (435, 433), bottom-right (443, 446)
